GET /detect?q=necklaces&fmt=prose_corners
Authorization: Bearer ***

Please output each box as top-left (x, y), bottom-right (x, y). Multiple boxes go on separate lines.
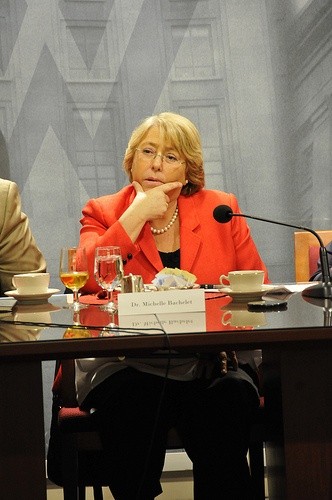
top-left (150, 207), bottom-right (178, 235)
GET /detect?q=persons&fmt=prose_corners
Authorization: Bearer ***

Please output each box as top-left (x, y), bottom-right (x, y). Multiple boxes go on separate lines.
top-left (0, 177), bottom-right (47, 297)
top-left (73, 112), bottom-right (271, 500)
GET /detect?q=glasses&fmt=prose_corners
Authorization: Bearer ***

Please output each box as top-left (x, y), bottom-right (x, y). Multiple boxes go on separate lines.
top-left (135, 147), bottom-right (186, 168)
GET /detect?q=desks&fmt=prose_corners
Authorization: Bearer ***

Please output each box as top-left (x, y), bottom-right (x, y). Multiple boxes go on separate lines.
top-left (1, 283), bottom-right (332, 500)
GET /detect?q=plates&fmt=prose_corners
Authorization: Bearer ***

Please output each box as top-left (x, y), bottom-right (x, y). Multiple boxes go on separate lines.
top-left (218, 286), bottom-right (270, 298)
top-left (11, 302), bottom-right (64, 314)
top-left (220, 301), bottom-right (249, 311)
top-left (4, 288), bottom-right (61, 300)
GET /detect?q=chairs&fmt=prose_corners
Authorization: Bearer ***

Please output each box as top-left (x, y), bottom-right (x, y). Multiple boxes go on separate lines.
top-left (48, 356), bottom-right (265, 500)
top-left (294, 230), bottom-right (332, 282)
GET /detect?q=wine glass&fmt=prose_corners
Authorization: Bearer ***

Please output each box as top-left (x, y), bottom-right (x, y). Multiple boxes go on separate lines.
top-left (59, 247), bottom-right (90, 307)
top-left (93, 246), bottom-right (125, 308)
top-left (99, 308), bottom-right (123, 337)
top-left (63, 307), bottom-right (91, 339)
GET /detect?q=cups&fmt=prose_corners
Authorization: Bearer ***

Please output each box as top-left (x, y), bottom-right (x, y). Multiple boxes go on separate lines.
top-left (219, 270), bottom-right (265, 291)
top-left (222, 311), bottom-right (267, 327)
top-left (11, 272), bottom-right (51, 294)
top-left (13, 313), bottom-right (52, 331)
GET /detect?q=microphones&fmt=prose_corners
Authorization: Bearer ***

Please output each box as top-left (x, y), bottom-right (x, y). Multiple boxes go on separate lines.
top-left (211, 205), bottom-right (332, 299)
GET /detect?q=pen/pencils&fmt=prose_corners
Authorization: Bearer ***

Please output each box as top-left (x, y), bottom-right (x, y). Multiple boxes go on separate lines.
top-left (200, 284), bottom-right (222, 289)
